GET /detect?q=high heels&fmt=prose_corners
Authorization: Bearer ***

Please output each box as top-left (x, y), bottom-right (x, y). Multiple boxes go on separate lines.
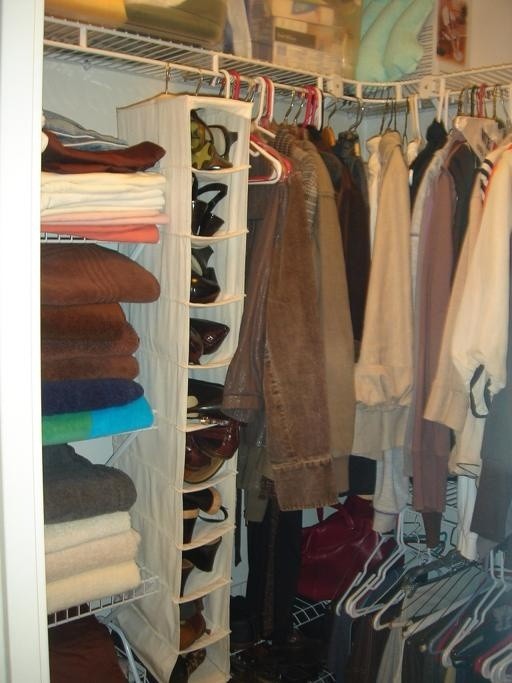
top-left (180, 110), bottom-right (240, 674)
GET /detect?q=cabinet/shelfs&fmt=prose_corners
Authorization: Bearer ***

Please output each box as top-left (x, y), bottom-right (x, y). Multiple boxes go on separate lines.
top-left (112, 94), bottom-right (255, 683)
top-left (231, 596), bottom-right (336, 683)
top-left (41, 231), bottom-right (162, 629)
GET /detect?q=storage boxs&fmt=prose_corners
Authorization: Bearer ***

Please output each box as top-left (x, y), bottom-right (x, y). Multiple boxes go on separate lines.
top-left (250, 17), bottom-right (345, 75)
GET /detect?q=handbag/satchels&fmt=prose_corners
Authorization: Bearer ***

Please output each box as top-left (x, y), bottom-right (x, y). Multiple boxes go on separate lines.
top-left (297, 495), bottom-right (405, 608)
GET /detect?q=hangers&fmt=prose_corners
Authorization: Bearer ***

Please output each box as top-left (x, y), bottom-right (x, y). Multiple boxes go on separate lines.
top-left (337, 506), bottom-right (512, 683)
